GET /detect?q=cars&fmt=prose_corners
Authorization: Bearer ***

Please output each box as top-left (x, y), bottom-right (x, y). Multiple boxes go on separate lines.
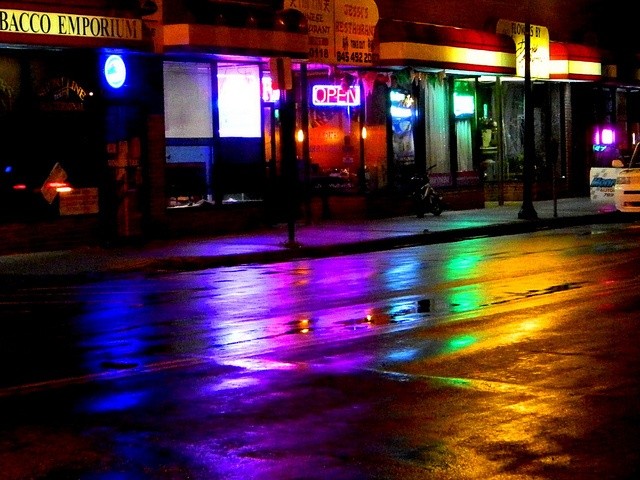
top-left (612, 142), bottom-right (640, 212)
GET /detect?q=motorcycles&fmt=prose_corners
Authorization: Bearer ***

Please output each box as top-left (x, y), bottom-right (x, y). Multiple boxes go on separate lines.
top-left (408, 164), bottom-right (442, 218)
